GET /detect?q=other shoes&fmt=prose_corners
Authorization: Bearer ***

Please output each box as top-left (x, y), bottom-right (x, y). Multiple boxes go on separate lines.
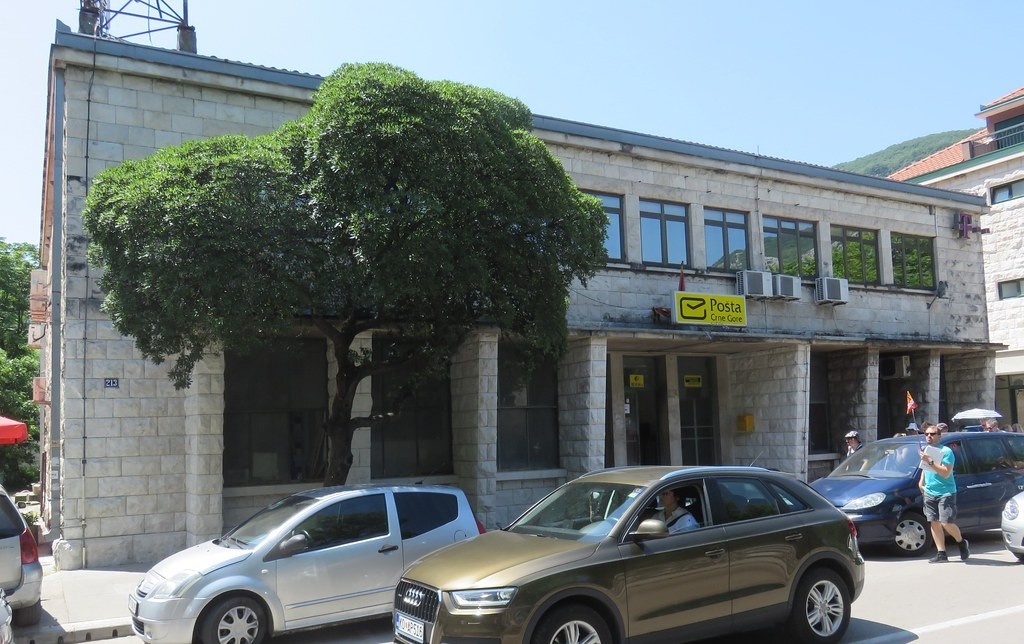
top-left (928, 553), bottom-right (948, 563)
top-left (960, 539), bottom-right (970, 560)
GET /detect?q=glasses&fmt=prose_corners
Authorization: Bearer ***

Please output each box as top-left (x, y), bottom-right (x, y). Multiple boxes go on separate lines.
top-left (924, 433), bottom-right (941, 436)
top-left (664, 490), bottom-right (673, 496)
top-left (986, 425), bottom-right (996, 429)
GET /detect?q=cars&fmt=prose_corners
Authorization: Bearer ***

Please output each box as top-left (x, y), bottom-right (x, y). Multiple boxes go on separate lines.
top-left (0, 480), bottom-right (42, 625)
top-left (392, 464), bottom-right (866, 644)
top-left (128, 478), bottom-right (487, 644)
top-left (1000, 489), bottom-right (1024, 563)
top-left (806, 431), bottom-right (1024, 557)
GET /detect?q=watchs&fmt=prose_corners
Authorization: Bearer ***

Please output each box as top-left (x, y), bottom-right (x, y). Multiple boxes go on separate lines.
top-left (929, 460), bottom-right (934, 466)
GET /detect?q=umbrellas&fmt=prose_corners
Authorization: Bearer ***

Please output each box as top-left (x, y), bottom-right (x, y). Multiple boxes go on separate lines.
top-left (951, 408), bottom-right (1002, 431)
top-left (0, 416), bottom-right (28, 445)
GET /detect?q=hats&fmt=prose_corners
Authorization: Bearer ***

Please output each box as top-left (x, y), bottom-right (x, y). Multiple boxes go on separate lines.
top-left (906, 423), bottom-right (919, 432)
top-left (845, 431), bottom-right (859, 438)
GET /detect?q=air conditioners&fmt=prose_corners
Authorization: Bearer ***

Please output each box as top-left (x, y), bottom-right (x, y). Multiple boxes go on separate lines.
top-left (30, 270), bottom-right (48, 321)
top-left (27, 324), bottom-right (48, 349)
top-left (33, 377), bottom-right (48, 401)
top-left (773, 274), bottom-right (802, 299)
top-left (815, 277), bottom-right (850, 304)
top-left (879, 355), bottom-right (911, 379)
top-left (735, 270), bottom-right (773, 300)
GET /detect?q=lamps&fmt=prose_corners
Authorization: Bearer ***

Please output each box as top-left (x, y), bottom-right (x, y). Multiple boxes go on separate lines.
top-left (927, 280), bottom-right (948, 309)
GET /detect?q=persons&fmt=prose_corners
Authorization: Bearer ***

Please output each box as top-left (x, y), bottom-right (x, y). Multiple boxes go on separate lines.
top-left (918, 426), bottom-right (970, 563)
top-left (845, 431), bottom-right (870, 471)
top-left (651, 487), bottom-right (700, 535)
top-left (884, 418), bottom-right (1023, 470)
